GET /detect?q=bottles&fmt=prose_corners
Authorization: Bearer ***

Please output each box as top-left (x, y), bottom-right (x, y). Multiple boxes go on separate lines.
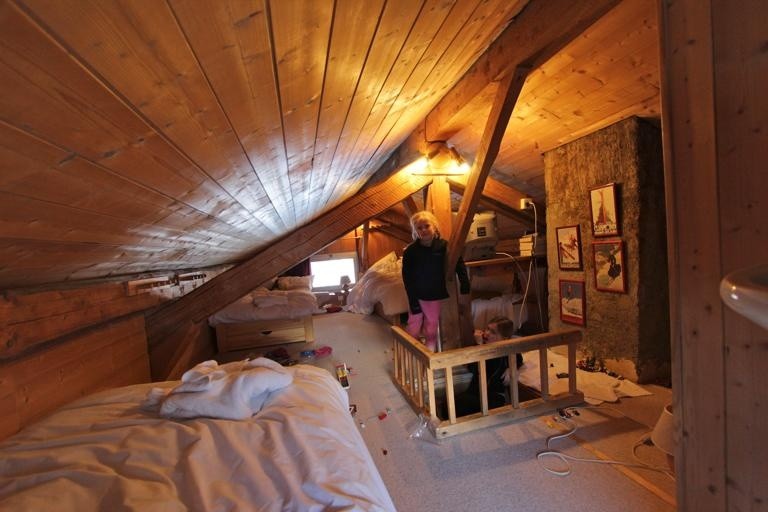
top-left (336, 364), bottom-right (351, 390)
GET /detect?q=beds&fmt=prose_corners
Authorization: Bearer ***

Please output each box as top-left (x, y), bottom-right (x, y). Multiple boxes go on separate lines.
top-left (1, 364), bottom-right (397, 511)
top-left (343, 276), bottom-right (407, 327)
top-left (208, 277), bottom-right (319, 355)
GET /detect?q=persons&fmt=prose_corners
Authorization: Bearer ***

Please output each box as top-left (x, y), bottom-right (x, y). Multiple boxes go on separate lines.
top-left (401, 211), bottom-right (470, 352)
top-left (461, 315), bottom-right (523, 416)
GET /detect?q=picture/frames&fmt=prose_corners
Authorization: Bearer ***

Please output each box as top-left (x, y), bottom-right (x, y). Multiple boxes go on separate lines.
top-left (552, 182), bottom-right (627, 326)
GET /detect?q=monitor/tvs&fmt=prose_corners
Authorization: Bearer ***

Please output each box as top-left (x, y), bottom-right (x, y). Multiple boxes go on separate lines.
top-left (450, 209), bottom-right (499, 261)
